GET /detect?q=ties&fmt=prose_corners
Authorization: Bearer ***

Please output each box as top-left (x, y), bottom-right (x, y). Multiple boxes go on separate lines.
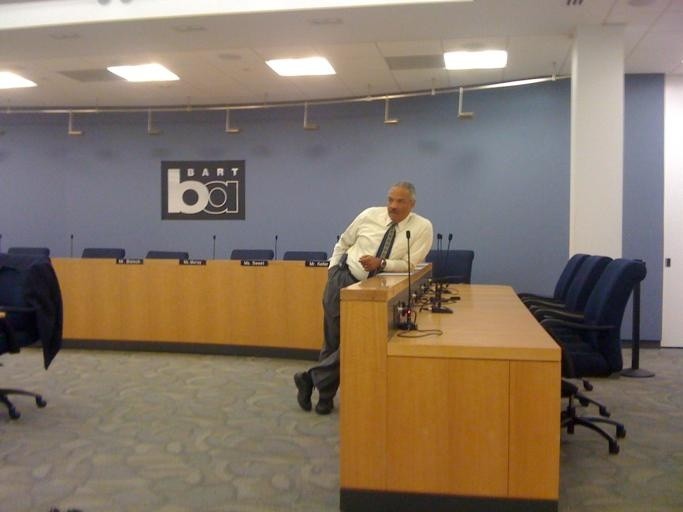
top-left (367, 222), bottom-right (396, 278)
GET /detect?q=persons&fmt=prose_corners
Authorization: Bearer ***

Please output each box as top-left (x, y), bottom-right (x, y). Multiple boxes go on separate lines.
top-left (294, 180), bottom-right (432, 413)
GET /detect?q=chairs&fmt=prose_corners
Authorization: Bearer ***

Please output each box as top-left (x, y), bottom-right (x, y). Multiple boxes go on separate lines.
top-left (81, 247), bottom-right (126, 260)
top-left (228, 248), bottom-right (274, 260)
top-left (536, 257), bottom-right (648, 454)
top-left (515, 253), bottom-right (589, 306)
top-left (525, 254), bottom-right (614, 321)
top-left (283, 250), bottom-right (327, 261)
top-left (425, 249), bottom-right (474, 285)
top-left (7, 246), bottom-right (49, 256)
top-left (0, 255), bottom-right (54, 420)
top-left (146, 250), bottom-right (188, 261)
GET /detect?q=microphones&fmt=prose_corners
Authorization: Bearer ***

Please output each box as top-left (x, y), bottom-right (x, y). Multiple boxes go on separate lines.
top-left (274, 234), bottom-right (278, 261)
top-left (213, 234), bottom-right (216, 260)
top-left (400, 231), bottom-right (418, 330)
top-left (430, 233), bottom-right (448, 302)
top-left (436, 232), bottom-right (453, 293)
top-left (432, 233), bottom-right (452, 314)
top-left (71, 234), bottom-right (74, 257)
top-left (336, 235), bottom-right (340, 242)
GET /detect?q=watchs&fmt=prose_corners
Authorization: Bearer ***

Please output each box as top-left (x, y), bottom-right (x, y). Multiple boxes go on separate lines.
top-left (379, 258), bottom-right (386, 272)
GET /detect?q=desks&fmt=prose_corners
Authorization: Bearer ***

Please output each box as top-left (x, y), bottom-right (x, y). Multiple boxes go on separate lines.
top-left (50, 257), bottom-right (328, 352)
top-left (338, 262), bottom-right (561, 512)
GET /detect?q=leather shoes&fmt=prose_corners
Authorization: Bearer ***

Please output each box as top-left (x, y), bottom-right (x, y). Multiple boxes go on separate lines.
top-left (294, 372), bottom-right (313, 412)
top-left (314, 396), bottom-right (335, 414)
